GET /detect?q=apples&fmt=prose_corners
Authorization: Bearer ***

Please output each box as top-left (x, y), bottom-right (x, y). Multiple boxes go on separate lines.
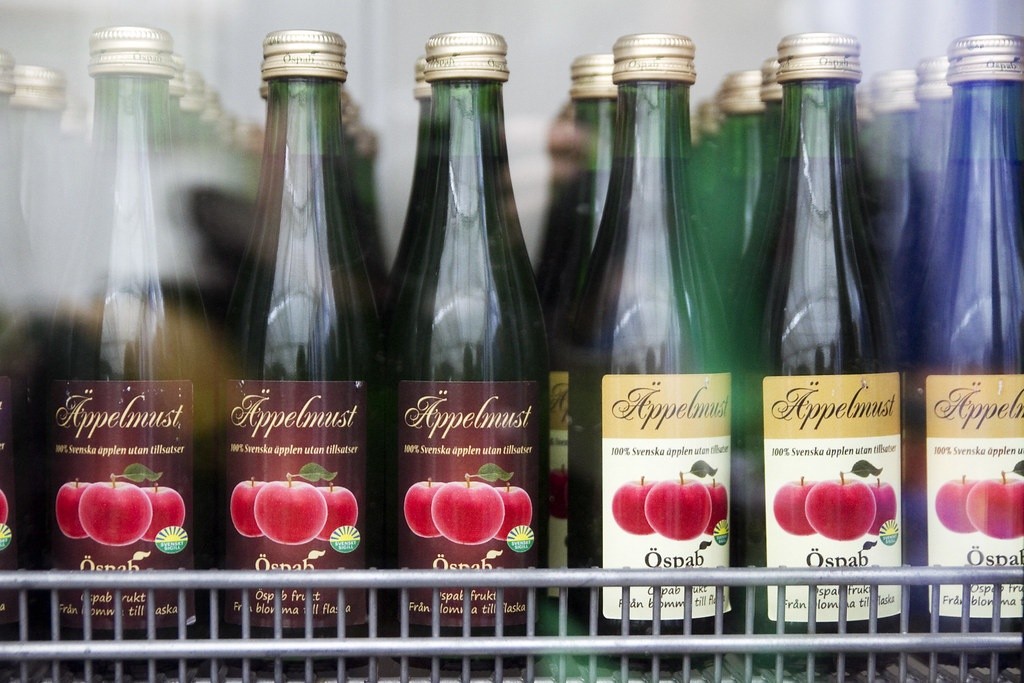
top-left (773, 471), bottom-right (898, 541)
top-left (612, 471), bottom-right (728, 541)
top-left (935, 471), bottom-right (1024, 539)
top-left (56, 472), bottom-right (185, 547)
top-left (403, 472), bottom-right (533, 545)
top-left (549, 464), bottom-right (569, 519)
top-left (0, 490), bottom-right (8, 524)
top-left (230, 471), bottom-right (359, 546)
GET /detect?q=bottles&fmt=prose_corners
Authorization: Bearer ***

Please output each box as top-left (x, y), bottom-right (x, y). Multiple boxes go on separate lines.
top-left (1, 25), bottom-right (1024, 669)
